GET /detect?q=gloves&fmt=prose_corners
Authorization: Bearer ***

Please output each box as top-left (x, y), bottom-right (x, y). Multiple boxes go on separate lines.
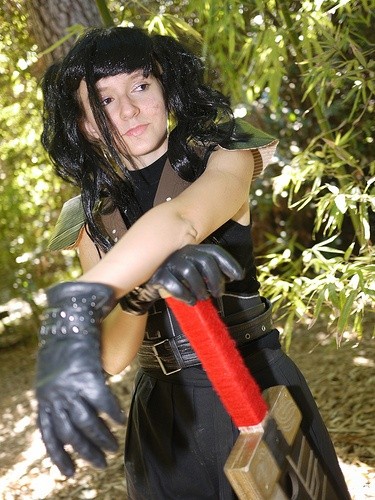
top-left (36, 282), bottom-right (129, 477)
top-left (150, 244), bottom-right (245, 306)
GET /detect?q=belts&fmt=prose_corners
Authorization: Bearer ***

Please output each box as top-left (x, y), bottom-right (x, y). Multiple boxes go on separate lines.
top-left (135, 296), bottom-right (272, 376)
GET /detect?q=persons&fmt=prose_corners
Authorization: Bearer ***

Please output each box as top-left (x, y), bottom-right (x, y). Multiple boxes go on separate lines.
top-left (28, 25), bottom-right (352, 500)
